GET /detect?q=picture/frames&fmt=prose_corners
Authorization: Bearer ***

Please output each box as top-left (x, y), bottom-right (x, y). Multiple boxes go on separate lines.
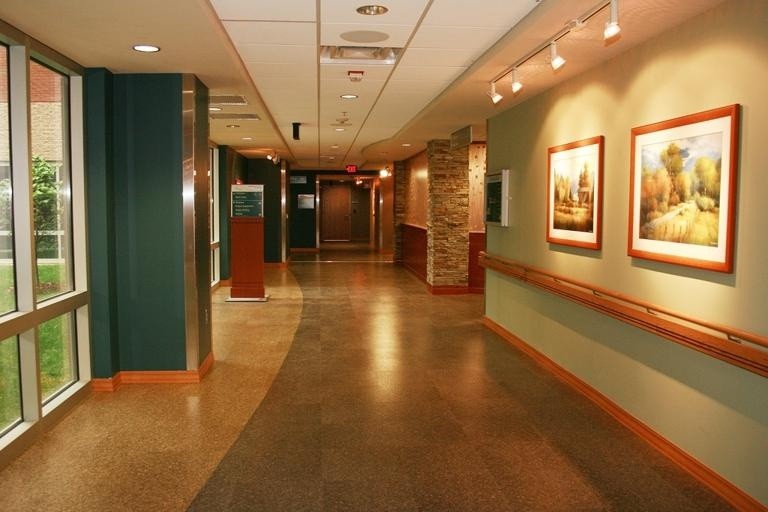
top-left (625, 103), bottom-right (740, 275)
top-left (547, 135), bottom-right (603, 252)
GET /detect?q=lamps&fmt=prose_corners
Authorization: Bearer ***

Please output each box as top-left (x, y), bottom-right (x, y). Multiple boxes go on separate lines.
top-left (487, 0), bottom-right (622, 105)
